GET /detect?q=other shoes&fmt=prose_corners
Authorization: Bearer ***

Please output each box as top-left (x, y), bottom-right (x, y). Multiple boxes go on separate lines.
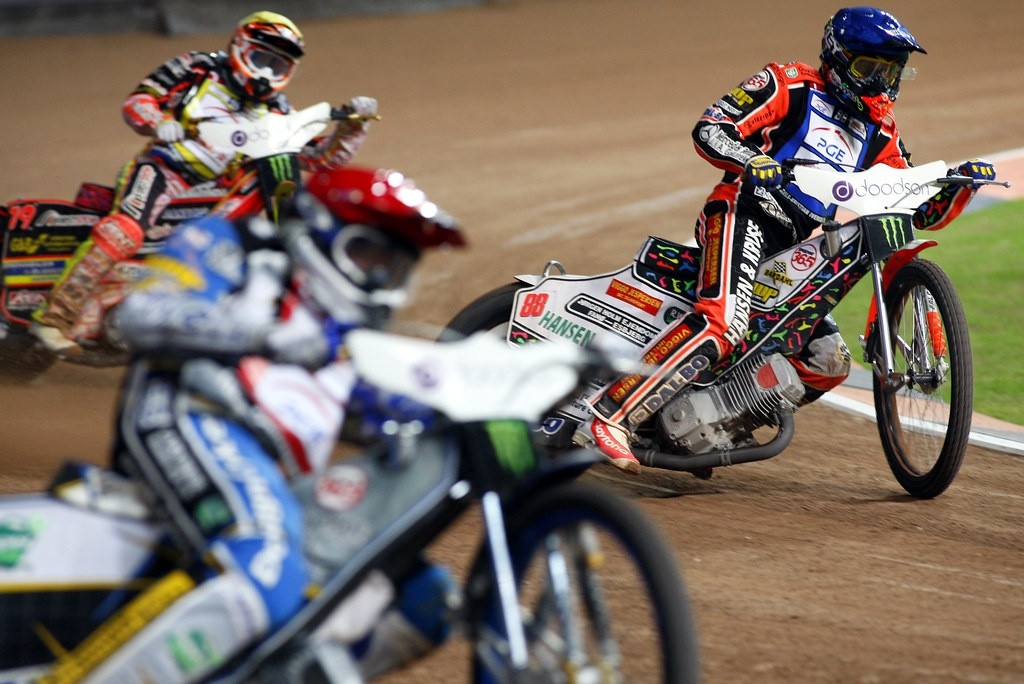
top-left (571, 413), bottom-right (644, 475)
top-left (26, 317), bottom-right (82, 359)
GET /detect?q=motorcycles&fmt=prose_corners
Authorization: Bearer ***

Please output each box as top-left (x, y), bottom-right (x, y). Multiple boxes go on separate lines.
top-left (0, 104), bottom-right (469, 386)
top-left (435, 177), bottom-right (1012, 498)
top-left (0, 325), bottom-right (701, 683)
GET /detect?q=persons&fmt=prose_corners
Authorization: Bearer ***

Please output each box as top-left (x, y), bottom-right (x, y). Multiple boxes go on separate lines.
top-left (28, 11), bottom-right (377, 351)
top-left (573, 7), bottom-right (995, 475)
top-left (25, 165), bottom-right (469, 684)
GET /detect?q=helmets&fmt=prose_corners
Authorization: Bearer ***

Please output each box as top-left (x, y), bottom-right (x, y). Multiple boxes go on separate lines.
top-left (283, 163), bottom-right (470, 323)
top-left (224, 10), bottom-right (307, 99)
top-left (819, 6), bottom-right (930, 118)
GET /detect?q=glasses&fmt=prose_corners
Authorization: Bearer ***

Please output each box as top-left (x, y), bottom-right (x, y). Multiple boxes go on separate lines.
top-left (849, 53), bottom-right (904, 87)
top-left (328, 223), bottom-right (417, 292)
top-left (245, 48), bottom-right (289, 78)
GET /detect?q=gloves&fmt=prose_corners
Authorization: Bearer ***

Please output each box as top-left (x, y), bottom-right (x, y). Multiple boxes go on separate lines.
top-left (745, 155), bottom-right (786, 191)
top-left (158, 119), bottom-right (184, 147)
top-left (351, 94), bottom-right (378, 120)
top-left (267, 320), bottom-right (332, 367)
top-left (948, 161), bottom-right (997, 191)
